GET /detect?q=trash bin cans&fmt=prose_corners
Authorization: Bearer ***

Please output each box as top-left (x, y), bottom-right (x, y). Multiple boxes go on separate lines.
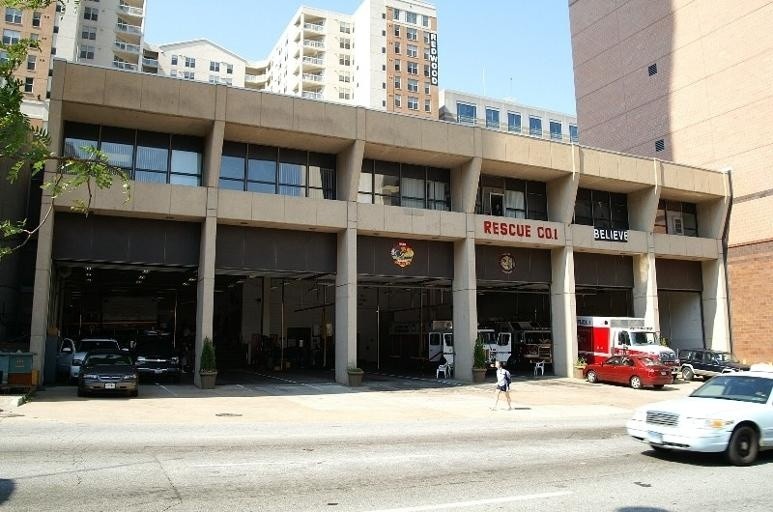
top-left (8, 350), bottom-right (33, 394)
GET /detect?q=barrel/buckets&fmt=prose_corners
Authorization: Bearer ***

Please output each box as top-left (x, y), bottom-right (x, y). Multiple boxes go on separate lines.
top-left (347, 371), bottom-right (365, 387)
top-left (201, 371), bottom-right (218, 388)
top-left (473, 368), bottom-right (487, 384)
top-left (574, 365), bottom-right (586, 378)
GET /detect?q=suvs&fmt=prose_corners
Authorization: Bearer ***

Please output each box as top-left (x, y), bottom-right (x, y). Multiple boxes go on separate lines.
top-left (676, 348), bottom-right (749, 382)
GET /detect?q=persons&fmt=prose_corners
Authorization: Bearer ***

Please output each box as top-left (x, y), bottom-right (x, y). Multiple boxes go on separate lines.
top-left (489, 359), bottom-right (512, 411)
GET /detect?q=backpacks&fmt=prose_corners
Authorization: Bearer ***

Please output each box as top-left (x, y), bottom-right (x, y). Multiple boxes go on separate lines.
top-left (506, 377), bottom-right (511, 385)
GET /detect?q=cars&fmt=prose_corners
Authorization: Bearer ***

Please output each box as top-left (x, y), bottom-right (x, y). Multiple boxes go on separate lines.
top-left (57, 331), bottom-right (183, 397)
top-left (626, 367), bottom-right (772, 462)
top-left (582, 354), bottom-right (674, 390)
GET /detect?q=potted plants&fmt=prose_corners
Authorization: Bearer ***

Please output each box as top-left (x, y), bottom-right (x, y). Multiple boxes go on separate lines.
top-left (573, 356), bottom-right (588, 379)
top-left (472, 334), bottom-right (488, 384)
top-left (346, 368), bottom-right (365, 386)
top-left (198, 335), bottom-right (219, 389)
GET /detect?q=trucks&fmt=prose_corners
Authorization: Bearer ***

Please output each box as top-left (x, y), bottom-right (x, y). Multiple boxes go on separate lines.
top-left (576, 315), bottom-right (681, 382)
top-left (389, 316), bottom-right (554, 373)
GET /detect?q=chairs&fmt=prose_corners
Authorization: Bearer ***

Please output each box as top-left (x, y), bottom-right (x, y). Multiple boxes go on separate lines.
top-left (533, 359), bottom-right (545, 377)
top-left (436, 360), bottom-right (449, 379)
